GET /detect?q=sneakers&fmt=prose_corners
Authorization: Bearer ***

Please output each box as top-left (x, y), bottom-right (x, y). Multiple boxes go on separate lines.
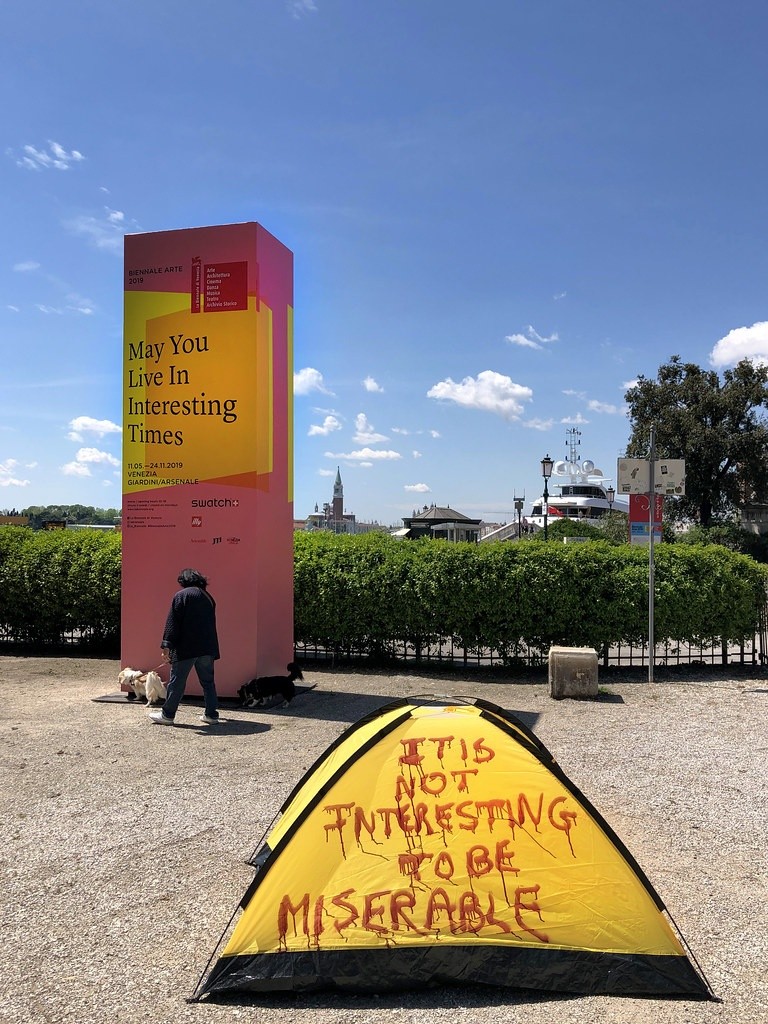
top-left (199, 714), bottom-right (219, 725)
top-left (148, 712), bottom-right (174, 725)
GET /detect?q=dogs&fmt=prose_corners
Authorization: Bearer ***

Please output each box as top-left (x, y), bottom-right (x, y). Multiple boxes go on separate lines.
top-left (237, 663), bottom-right (304, 708)
top-left (117, 666), bottom-right (167, 708)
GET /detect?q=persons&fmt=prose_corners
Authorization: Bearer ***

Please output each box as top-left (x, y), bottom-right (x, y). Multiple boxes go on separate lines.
top-left (151, 568), bottom-right (221, 725)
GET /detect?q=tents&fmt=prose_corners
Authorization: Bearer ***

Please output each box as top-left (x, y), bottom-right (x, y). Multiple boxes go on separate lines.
top-left (184, 693), bottom-right (722, 1005)
